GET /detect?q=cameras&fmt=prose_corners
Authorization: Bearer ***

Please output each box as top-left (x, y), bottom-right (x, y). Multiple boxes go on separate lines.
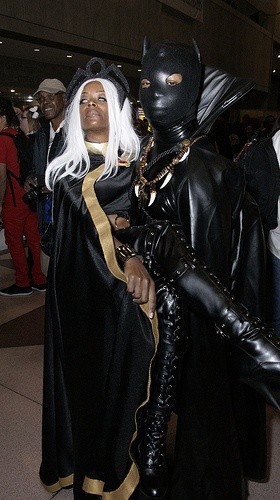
top-left (23, 185), bottom-right (49, 203)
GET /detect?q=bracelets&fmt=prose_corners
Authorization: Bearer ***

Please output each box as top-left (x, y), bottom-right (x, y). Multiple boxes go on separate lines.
top-left (114, 244), bottom-right (135, 266)
top-left (114, 216), bottom-right (119, 229)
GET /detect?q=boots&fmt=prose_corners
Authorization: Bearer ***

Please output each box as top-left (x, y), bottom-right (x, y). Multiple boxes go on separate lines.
top-left (136, 283), bottom-right (183, 500)
top-left (145, 221), bottom-right (280, 411)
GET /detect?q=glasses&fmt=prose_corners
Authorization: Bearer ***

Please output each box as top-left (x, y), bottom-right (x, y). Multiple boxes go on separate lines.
top-left (36, 94), bottom-right (63, 103)
top-left (21, 116), bottom-right (27, 120)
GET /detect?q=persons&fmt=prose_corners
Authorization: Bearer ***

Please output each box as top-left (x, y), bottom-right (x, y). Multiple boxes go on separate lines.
top-left (40, 38), bottom-right (280, 500)
top-left (0, 79), bottom-right (67, 296)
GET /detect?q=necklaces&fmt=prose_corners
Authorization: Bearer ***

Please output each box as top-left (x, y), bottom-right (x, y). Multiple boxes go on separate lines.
top-left (138, 134), bottom-right (193, 202)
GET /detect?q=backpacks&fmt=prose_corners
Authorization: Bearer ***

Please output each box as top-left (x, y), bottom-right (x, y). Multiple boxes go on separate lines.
top-left (0, 128), bottom-right (34, 187)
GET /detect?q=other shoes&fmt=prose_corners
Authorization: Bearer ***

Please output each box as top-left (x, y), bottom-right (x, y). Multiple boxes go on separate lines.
top-left (0, 283), bottom-right (32, 296)
top-left (30, 281), bottom-right (46, 291)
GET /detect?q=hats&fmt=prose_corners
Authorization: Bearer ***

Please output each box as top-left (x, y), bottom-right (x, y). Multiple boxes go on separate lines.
top-left (33, 78), bottom-right (66, 97)
